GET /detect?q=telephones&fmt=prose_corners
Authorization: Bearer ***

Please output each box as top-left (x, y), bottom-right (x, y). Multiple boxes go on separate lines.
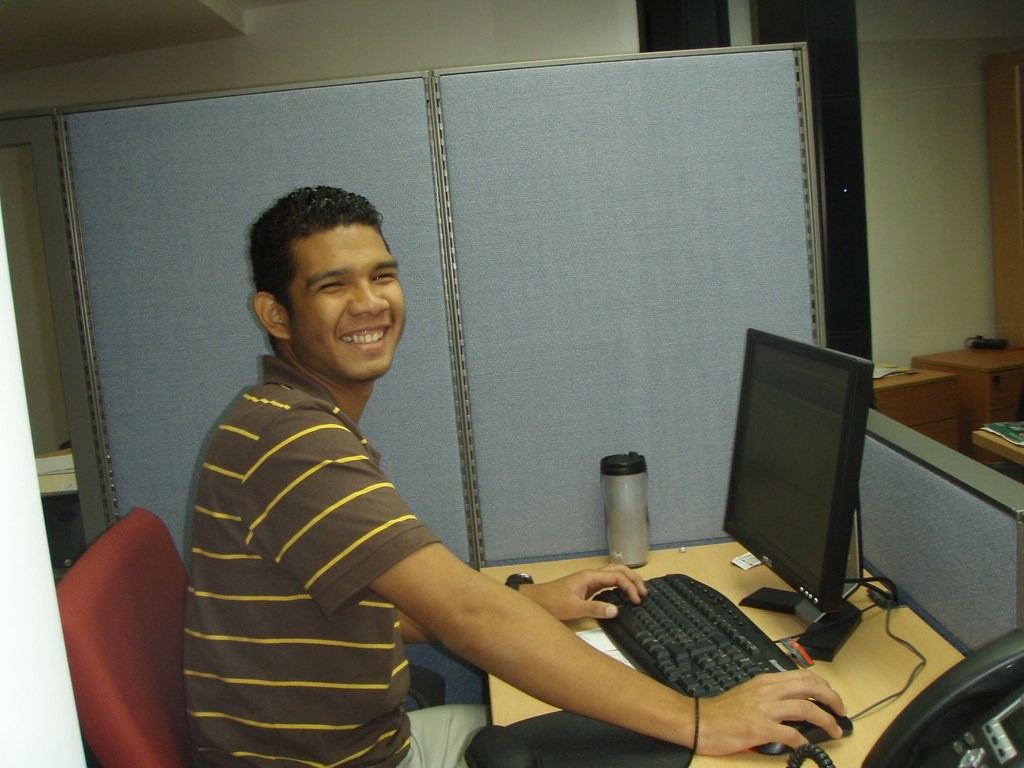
top-left (859, 627), bottom-right (1024, 768)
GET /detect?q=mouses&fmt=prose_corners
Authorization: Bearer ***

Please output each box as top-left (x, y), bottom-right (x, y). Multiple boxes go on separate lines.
top-left (757, 700), bottom-right (852, 756)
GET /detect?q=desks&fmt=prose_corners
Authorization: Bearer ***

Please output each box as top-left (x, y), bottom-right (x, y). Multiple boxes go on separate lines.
top-left (473, 540), bottom-right (974, 768)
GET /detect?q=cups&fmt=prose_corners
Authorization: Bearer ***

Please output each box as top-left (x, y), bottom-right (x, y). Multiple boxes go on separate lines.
top-left (601, 451), bottom-right (651, 569)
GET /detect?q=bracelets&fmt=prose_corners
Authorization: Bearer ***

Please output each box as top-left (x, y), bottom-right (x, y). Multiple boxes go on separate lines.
top-left (691, 695), bottom-right (699, 754)
top-left (505, 573), bottom-right (533, 591)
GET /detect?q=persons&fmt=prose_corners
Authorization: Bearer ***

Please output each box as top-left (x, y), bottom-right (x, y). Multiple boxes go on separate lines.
top-left (184, 185), bottom-right (849, 768)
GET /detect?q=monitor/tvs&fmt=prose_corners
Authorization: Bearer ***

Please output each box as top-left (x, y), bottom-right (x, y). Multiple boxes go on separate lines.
top-left (720, 327), bottom-right (876, 662)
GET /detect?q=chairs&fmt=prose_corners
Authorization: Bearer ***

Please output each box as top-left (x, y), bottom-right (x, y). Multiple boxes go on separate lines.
top-left (50, 501), bottom-right (202, 768)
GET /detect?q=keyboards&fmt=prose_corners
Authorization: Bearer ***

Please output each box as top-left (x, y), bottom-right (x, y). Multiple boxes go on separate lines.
top-left (596, 574), bottom-right (800, 698)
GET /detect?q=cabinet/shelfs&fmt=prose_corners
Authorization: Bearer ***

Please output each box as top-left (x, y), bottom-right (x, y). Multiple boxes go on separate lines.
top-left (874, 365), bottom-right (961, 449)
top-left (911, 348), bottom-right (1022, 437)
